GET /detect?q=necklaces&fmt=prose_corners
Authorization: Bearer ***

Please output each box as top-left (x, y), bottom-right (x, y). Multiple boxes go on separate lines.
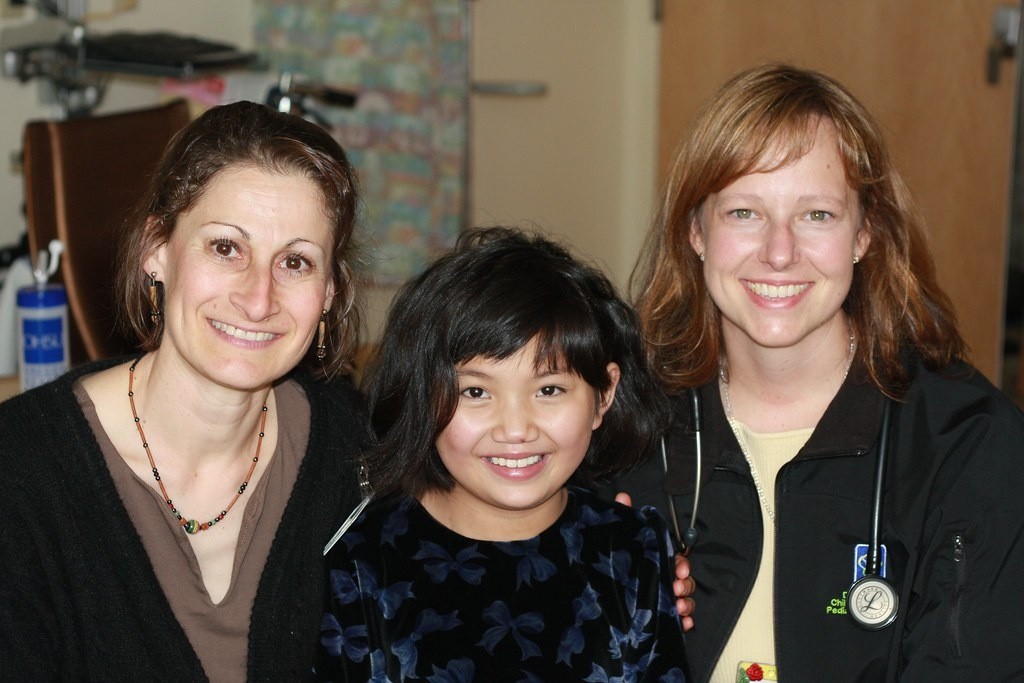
top-left (718, 316), bottom-right (855, 522)
top-left (129, 350), bottom-right (268, 533)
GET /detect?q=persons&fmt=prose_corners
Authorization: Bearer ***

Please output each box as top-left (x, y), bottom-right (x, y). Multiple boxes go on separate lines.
top-left (626, 65), bottom-right (1024, 683)
top-left (0, 101), bottom-right (690, 683)
top-left (321, 226), bottom-right (692, 683)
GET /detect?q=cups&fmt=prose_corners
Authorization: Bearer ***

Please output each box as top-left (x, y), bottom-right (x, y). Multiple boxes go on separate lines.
top-left (16, 285), bottom-right (70, 395)
